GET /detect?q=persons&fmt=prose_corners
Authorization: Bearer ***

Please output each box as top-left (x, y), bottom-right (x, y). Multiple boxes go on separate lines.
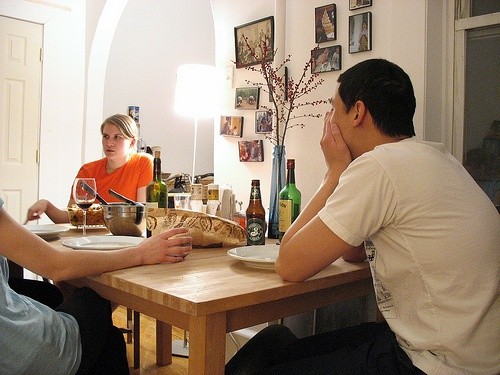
top-left (223, 22), bottom-right (368, 162)
top-left (223, 59), bottom-right (500, 375)
top-left (0, 199), bottom-right (193, 375)
top-left (23, 113), bottom-right (152, 226)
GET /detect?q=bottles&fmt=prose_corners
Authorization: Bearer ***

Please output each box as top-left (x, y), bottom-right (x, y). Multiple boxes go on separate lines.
top-left (279, 158), bottom-right (302, 243)
top-left (145, 150), bottom-right (168, 239)
top-left (246, 180), bottom-right (265, 246)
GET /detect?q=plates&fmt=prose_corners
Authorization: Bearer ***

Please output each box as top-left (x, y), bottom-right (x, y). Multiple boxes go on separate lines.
top-left (22, 225), bottom-right (70, 239)
top-left (62, 236), bottom-right (147, 250)
top-left (227, 244), bottom-right (281, 270)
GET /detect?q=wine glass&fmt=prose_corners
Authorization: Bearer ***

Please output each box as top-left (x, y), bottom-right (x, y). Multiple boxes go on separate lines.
top-left (72, 178), bottom-right (97, 236)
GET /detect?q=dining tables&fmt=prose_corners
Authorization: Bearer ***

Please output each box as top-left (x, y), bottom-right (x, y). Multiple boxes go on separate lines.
top-left (43, 229), bottom-right (384, 375)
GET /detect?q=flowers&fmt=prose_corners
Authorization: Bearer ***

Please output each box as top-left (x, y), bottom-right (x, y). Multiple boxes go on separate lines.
top-left (231, 30), bottom-right (326, 222)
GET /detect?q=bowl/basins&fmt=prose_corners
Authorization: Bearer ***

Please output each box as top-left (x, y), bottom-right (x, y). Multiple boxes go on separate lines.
top-left (101, 203), bottom-right (146, 237)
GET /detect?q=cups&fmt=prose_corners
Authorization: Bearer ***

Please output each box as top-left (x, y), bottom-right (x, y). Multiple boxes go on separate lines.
top-left (174, 184), bottom-right (221, 216)
top-left (159, 220), bottom-right (193, 263)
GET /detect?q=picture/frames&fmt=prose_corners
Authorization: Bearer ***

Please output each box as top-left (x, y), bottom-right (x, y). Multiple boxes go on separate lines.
top-left (268, 65), bottom-right (288, 101)
top-left (234, 15), bottom-right (275, 68)
top-left (348, 0), bottom-right (372, 10)
top-left (234, 87), bottom-right (259, 110)
top-left (314, 3), bottom-right (337, 42)
top-left (237, 140), bottom-right (264, 162)
top-left (348, 12), bottom-right (372, 54)
top-left (255, 110), bottom-right (272, 133)
top-left (311, 45), bottom-right (342, 74)
top-left (219, 115), bottom-right (243, 137)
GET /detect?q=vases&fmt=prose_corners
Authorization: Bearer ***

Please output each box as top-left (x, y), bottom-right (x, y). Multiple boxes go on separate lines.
top-left (268, 145), bottom-right (286, 239)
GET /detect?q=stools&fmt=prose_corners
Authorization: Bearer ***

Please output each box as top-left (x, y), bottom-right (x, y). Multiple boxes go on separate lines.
top-left (115, 309), bottom-right (141, 369)
